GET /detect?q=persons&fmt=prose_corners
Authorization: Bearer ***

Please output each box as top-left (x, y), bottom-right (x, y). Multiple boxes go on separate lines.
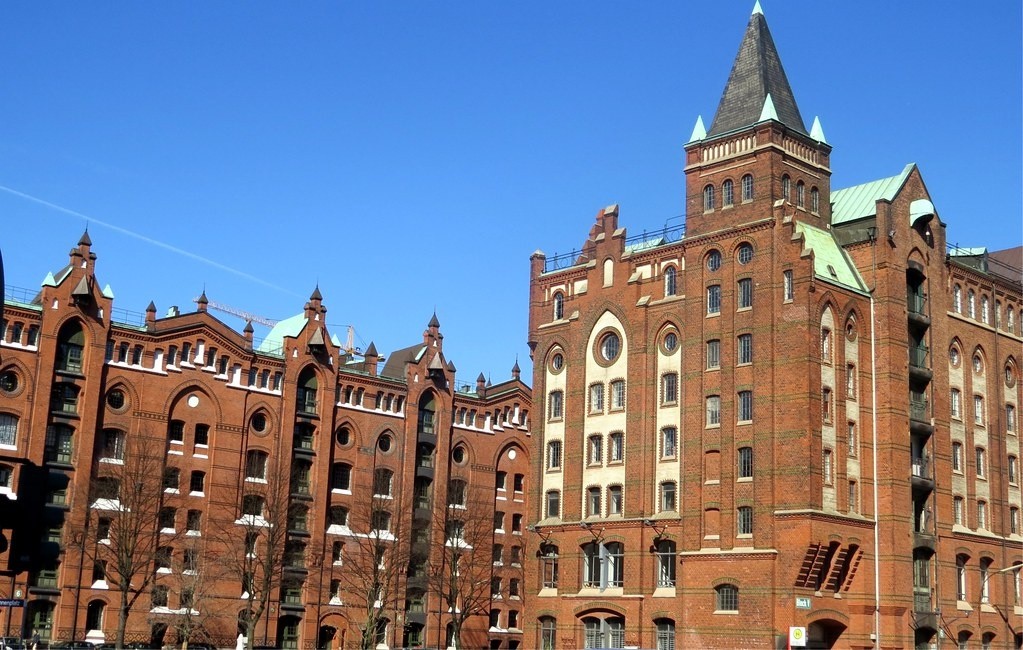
top-left (30, 629), bottom-right (41, 650)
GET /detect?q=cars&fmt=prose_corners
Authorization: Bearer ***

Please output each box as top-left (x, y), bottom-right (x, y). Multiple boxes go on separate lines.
top-left (124, 642), bottom-right (161, 650)
top-left (94, 643), bottom-right (126, 650)
top-left (188, 642), bottom-right (217, 650)
top-left (50, 640), bottom-right (95, 650)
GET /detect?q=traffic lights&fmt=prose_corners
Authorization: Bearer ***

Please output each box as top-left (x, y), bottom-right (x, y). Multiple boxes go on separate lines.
top-left (8, 461), bottom-right (71, 575)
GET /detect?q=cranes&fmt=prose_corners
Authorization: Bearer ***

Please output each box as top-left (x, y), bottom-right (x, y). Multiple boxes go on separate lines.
top-left (192, 296), bottom-right (386, 362)
